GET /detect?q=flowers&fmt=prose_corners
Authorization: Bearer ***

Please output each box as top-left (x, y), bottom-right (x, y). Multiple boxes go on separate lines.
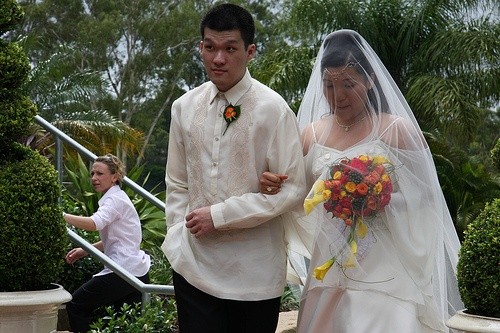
top-left (218, 102), bottom-right (244, 136)
top-left (303, 152), bottom-right (393, 280)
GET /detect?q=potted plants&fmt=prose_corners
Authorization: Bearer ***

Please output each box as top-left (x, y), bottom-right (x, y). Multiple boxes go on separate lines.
top-left (0, 39), bottom-right (73, 333)
top-left (446, 198), bottom-right (500, 333)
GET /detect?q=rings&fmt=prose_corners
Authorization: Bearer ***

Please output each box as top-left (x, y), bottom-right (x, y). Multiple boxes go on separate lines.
top-left (266, 187), bottom-right (272, 191)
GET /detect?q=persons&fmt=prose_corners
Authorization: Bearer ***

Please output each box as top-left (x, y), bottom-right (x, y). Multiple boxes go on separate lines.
top-left (162, 4), bottom-right (307, 333)
top-left (63, 154), bottom-right (151, 333)
top-left (260, 29), bottom-right (464, 332)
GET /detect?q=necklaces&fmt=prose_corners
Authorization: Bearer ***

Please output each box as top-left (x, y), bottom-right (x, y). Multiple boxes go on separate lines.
top-left (335, 113), bottom-right (369, 131)
